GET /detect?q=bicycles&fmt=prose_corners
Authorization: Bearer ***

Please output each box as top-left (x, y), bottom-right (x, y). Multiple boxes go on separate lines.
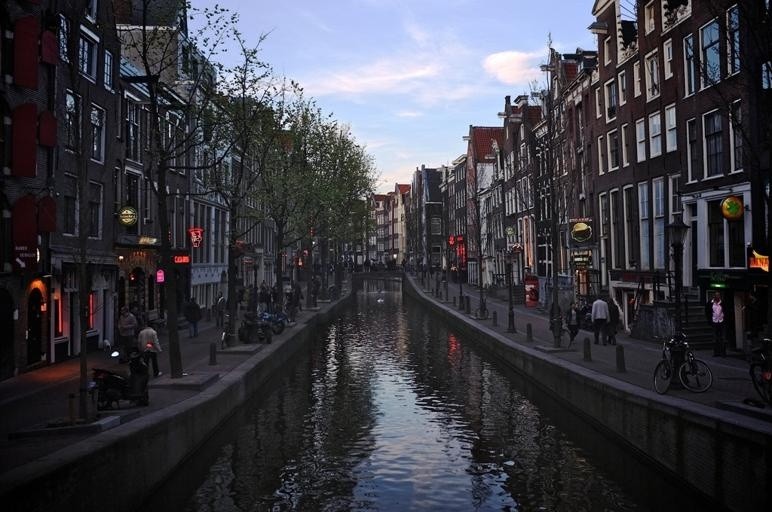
top-left (474, 289), bottom-right (490, 321)
top-left (653, 334), bottom-right (678, 396)
top-left (675, 329), bottom-right (714, 394)
top-left (749, 338), bottom-right (772, 407)
top-left (549, 314), bottom-right (572, 349)
top-left (221, 314), bottom-right (232, 350)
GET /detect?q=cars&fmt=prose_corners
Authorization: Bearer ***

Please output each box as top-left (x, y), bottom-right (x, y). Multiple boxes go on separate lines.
top-left (273, 276), bottom-right (296, 294)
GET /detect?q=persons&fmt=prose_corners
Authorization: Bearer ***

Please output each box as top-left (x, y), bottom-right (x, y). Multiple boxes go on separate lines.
top-left (667, 314), bottom-right (687, 381)
top-left (137, 322), bottom-right (162, 377)
top-left (704, 290), bottom-right (729, 357)
top-left (117, 306), bottom-right (138, 364)
top-left (184, 298), bottom-right (201, 337)
top-left (215, 250), bottom-right (440, 327)
top-left (549, 295), bottom-right (618, 346)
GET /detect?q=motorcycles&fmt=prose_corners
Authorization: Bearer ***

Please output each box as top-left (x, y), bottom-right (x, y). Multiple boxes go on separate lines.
top-left (90, 338), bottom-right (155, 412)
top-left (260, 309), bottom-right (286, 335)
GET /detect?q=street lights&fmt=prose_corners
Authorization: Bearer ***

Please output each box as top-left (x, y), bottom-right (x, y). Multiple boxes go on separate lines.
top-left (666, 212), bottom-right (691, 390)
top-left (504, 249), bottom-right (516, 334)
top-left (245, 253), bottom-right (260, 342)
top-left (456, 255), bottom-right (464, 310)
